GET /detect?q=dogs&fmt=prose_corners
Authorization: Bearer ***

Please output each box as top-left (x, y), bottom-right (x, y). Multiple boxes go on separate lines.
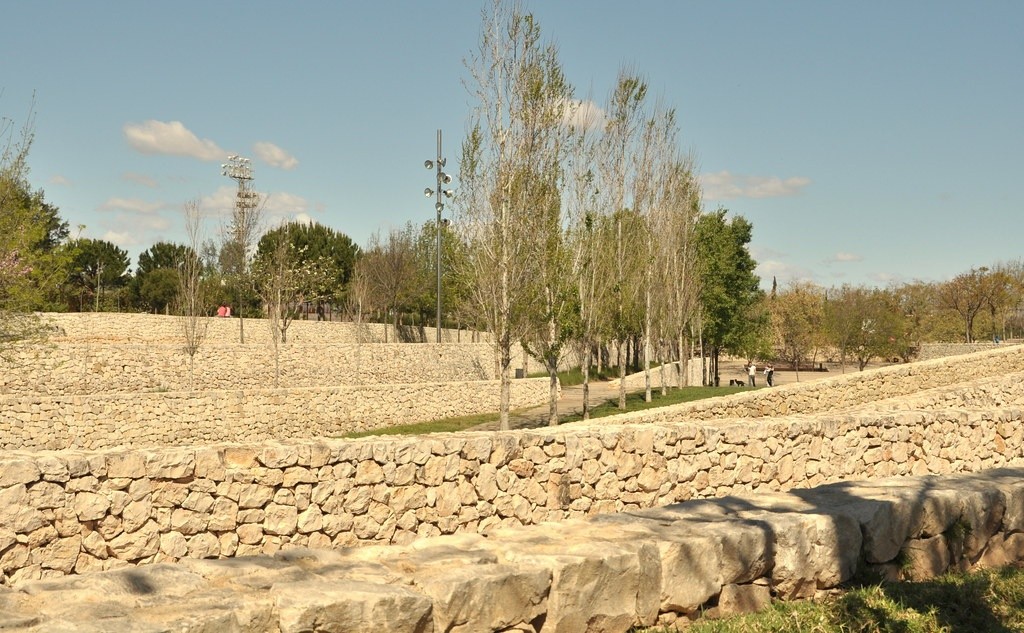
top-left (735, 379), bottom-right (747, 388)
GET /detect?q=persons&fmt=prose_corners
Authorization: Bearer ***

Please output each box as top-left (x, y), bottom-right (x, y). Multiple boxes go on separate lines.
top-left (995, 335), bottom-right (999, 344)
top-left (745, 361), bottom-right (756, 388)
top-left (316, 301), bottom-right (325, 322)
top-left (215, 303), bottom-right (231, 318)
top-left (764, 362), bottom-right (774, 387)
